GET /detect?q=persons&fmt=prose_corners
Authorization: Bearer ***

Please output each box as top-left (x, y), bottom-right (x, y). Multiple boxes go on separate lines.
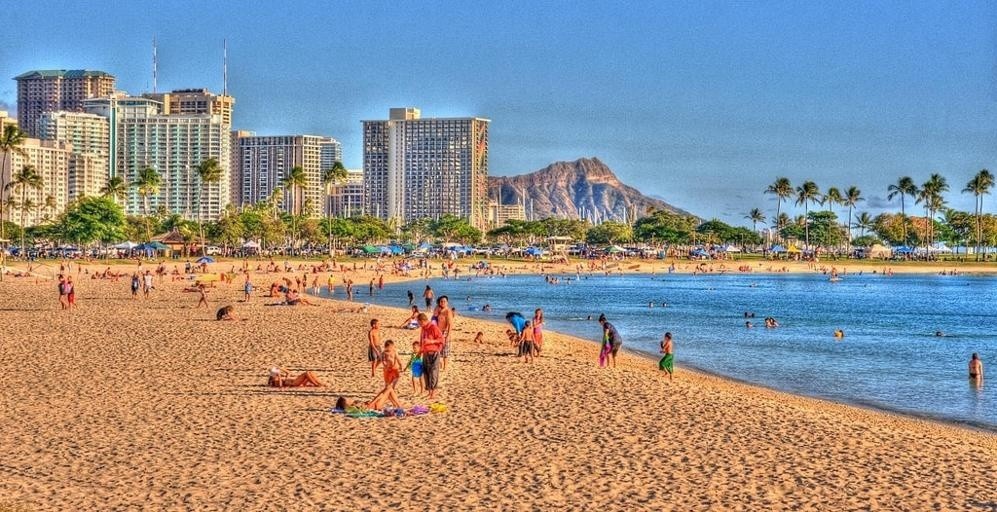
top-left (368, 319), bottom-right (383, 377)
top-left (598, 313), bottom-right (623, 367)
top-left (402, 341), bottom-right (425, 393)
top-left (437, 296), bottom-right (454, 370)
top-left (695, 260), bottom-right (751, 274)
top-left (506, 308), bottom-right (544, 364)
top-left (774, 259), bottom-right (895, 282)
top-left (938, 268), bottom-right (959, 275)
top-left (442, 258), bottom-right (608, 285)
top-left (382, 340), bottom-right (404, 407)
top-left (474, 332), bottom-right (484, 343)
top-left (660, 332), bottom-right (674, 379)
top-left (742, 312), bottom-right (779, 328)
top-left (416, 313), bottom-right (445, 398)
top-left (969, 353), bottom-right (983, 388)
top-left (431, 297), bottom-right (442, 325)
top-left (268, 366), bottom-right (325, 387)
